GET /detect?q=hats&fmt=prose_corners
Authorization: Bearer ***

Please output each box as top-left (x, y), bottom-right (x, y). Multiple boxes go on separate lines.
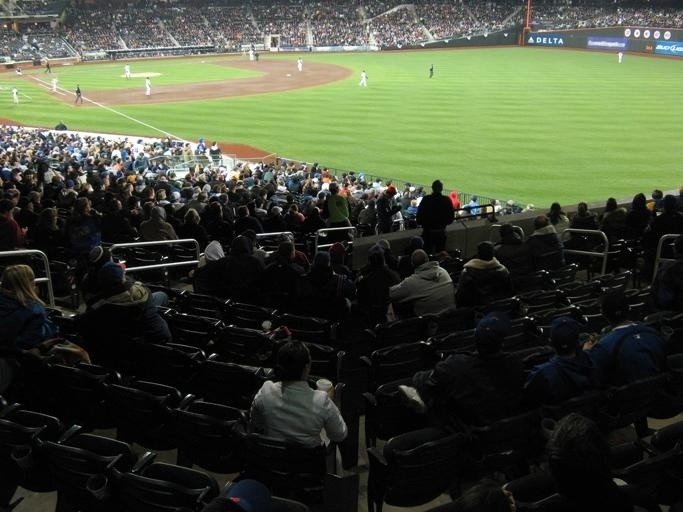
top-left (271, 340), bottom-right (312, 385)
top-left (223, 478), bottom-right (271, 512)
top-left (89, 246), bottom-right (111, 267)
top-left (477, 312), bottom-right (505, 351)
top-left (552, 315), bottom-right (579, 343)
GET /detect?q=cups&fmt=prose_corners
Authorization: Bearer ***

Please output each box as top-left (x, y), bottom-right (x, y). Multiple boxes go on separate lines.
top-left (315, 379), bottom-right (333, 393)
top-left (261, 320), bottom-right (271, 329)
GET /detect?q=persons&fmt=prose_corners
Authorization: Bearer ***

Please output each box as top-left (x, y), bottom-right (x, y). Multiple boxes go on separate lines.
top-left (74, 84), bottom-right (82, 104)
top-left (0, 122), bottom-right (681, 512)
top-left (12, 87), bottom-right (18, 105)
top-left (51, 77), bottom-right (58, 93)
top-left (145, 77), bottom-right (151, 95)
top-left (358, 70), bottom-right (367, 87)
top-left (0, 0), bottom-right (683, 78)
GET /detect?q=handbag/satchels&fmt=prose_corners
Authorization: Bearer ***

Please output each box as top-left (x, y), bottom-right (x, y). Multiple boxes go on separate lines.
top-left (542, 412), bottom-right (583, 453)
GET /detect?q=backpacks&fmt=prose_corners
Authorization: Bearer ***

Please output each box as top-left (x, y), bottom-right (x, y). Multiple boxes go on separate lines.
top-left (32, 337), bottom-right (91, 367)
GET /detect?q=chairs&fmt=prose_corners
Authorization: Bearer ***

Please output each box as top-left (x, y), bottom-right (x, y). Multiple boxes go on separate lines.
top-left (0, 185), bottom-right (683, 511)
